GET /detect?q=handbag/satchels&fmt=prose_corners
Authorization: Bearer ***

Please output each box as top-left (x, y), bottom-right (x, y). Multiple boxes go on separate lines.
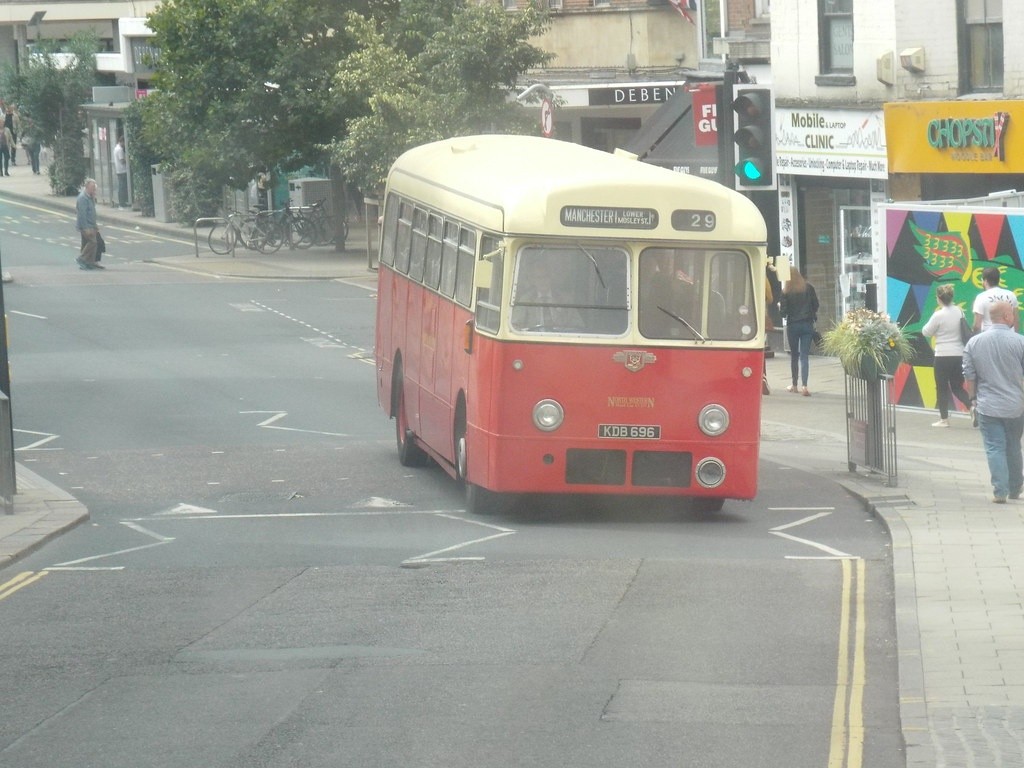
top-left (812, 331), bottom-right (824, 346)
top-left (959, 309), bottom-right (973, 345)
top-left (765, 316), bottom-right (774, 333)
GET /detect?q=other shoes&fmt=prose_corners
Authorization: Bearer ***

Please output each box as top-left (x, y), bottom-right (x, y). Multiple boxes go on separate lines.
top-left (932, 420), bottom-right (949, 427)
top-left (993, 497), bottom-right (1007, 503)
top-left (970, 406), bottom-right (978, 427)
top-left (1010, 485), bottom-right (1024, 498)
top-left (801, 388), bottom-right (809, 395)
top-left (76, 256), bottom-right (89, 270)
top-left (788, 385), bottom-right (798, 393)
top-left (762, 377), bottom-right (770, 395)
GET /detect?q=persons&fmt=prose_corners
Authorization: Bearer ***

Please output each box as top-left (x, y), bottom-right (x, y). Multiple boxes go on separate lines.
top-left (114, 136), bottom-right (130, 209)
top-left (761, 272), bottom-right (772, 394)
top-left (778, 266), bottom-right (819, 396)
top-left (0, 106), bottom-right (41, 176)
top-left (922, 283), bottom-right (978, 428)
top-left (962, 300), bottom-right (1024, 503)
top-left (972, 267), bottom-right (1019, 334)
top-left (75, 178), bottom-right (105, 272)
top-left (511, 268), bottom-right (587, 332)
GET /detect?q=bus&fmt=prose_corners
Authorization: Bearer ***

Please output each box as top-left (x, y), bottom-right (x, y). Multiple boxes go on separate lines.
top-left (373, 134), bottom-right (769, 517)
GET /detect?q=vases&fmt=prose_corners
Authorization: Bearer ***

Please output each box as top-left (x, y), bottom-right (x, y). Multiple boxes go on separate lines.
top-left (837, 348), bottom-right (901, 382)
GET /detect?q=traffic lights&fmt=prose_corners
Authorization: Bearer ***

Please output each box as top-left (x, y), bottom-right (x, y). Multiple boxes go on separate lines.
top-left (728, 83), bottom-right (777, 194)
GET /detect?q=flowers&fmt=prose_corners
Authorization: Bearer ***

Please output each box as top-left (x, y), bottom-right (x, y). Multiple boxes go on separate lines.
top-left (816, 306), bottom-right (920, 381)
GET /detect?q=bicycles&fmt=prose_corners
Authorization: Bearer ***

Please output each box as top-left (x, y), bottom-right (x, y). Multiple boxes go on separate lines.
top-left (209, 198), bottom-right (351, 255)
